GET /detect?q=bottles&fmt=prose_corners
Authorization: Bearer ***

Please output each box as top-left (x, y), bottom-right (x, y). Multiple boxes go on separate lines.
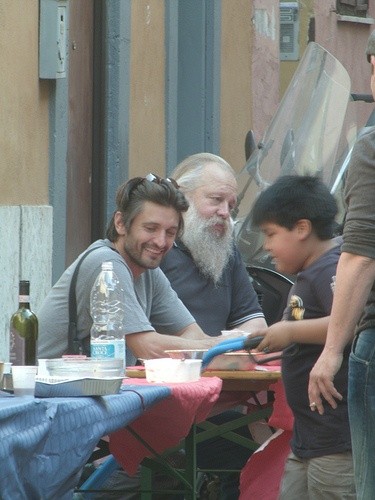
top-left (89, 262), bottom-right (124, 358)
top-left (9, 280), bottom-right (38, 366)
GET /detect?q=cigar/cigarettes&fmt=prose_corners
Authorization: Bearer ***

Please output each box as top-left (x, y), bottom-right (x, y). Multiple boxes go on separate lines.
top-left (310, 402), bottom-right (316, 407)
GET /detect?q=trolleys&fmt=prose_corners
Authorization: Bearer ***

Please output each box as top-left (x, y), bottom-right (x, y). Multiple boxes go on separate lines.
top-left (71, 335), bottom-right (301, 500)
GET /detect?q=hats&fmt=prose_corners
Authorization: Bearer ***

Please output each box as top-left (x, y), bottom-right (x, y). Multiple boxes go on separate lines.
top-left (366, 29), bottom-right (375, 64)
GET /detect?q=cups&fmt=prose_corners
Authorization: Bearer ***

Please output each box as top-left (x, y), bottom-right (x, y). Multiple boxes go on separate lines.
top-left (220, 330), bottom-right (244, 336)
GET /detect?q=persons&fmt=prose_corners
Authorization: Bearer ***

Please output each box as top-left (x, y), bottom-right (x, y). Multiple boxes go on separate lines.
top-left (308, 28), bottom-right (375, 500)
top-left (160, 153), bottom-right (268, 342)
top-left (244, 173), bottom-right (355, 500)
top-left (35, 173), bottom-right (246, 366)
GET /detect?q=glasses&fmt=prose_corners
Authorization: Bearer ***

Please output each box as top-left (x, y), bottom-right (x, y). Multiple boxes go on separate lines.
top-left (146, 172), bottom-right (178, 188)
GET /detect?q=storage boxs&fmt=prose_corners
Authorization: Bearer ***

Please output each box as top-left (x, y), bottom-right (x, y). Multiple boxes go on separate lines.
top-left (145, 357), bottom-right (202, 383)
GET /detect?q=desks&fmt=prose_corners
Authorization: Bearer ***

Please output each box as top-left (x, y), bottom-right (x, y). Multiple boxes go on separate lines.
top-left (0, 364), bottom-right (282, 500)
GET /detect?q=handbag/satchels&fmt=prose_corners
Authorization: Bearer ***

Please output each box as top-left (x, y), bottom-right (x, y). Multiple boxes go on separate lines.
top-left (96, 467), bottom-right (179, 499)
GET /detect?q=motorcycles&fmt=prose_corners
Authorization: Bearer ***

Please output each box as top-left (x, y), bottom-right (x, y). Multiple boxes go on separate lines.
top-left (230, 40), bottom-right (375, 327)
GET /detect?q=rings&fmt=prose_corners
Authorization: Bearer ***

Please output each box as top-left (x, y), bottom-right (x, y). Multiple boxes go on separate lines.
top-left (264, 347), bottom-right (271, 353)
